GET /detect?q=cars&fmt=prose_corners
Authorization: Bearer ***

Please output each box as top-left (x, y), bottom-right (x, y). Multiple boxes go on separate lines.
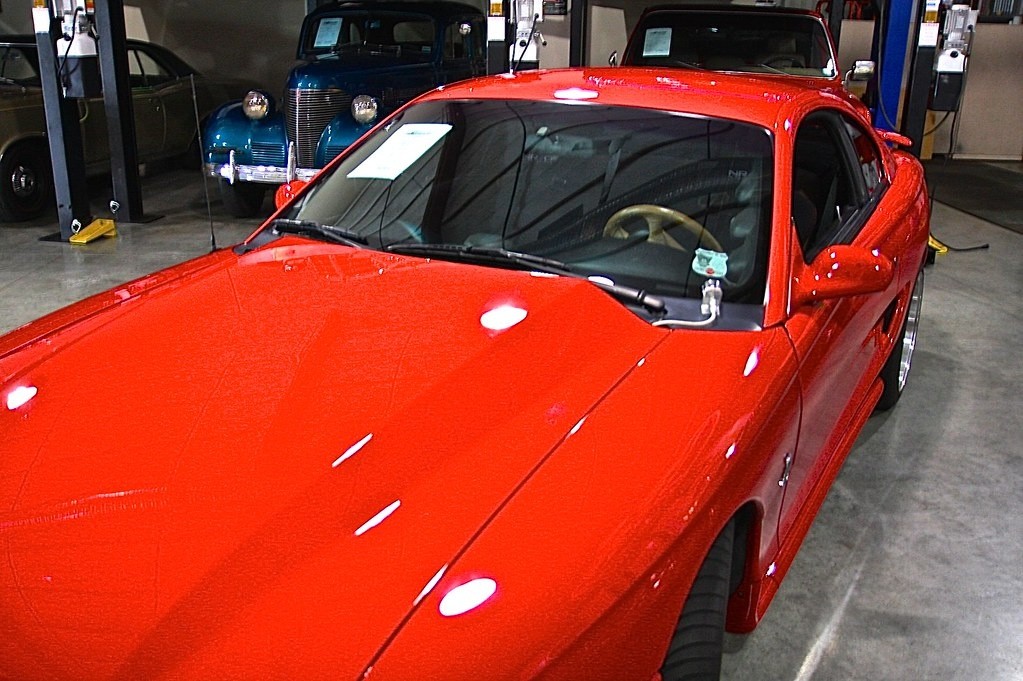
top-left (200, 0), bottom-right (494, 219)
top-left (1, 37), bottom-right (275, 218)
top-left (607, 5), bottom-right (877, 129)
top-left (1, 67), bottom-right (931, 681)
